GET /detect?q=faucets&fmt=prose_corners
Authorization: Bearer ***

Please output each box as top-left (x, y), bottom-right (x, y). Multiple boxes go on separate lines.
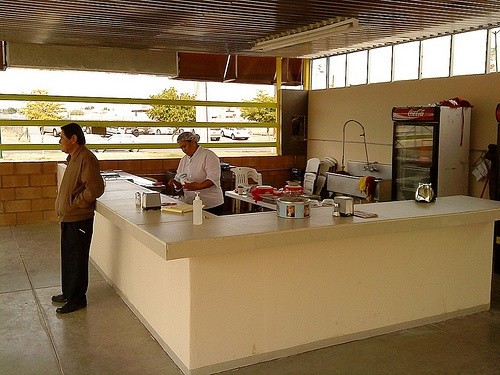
top-left (341, 119), bottom-right (371, 170)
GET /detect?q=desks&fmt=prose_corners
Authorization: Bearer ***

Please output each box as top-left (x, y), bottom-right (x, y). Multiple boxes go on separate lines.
top-left (225, 190), bottom-right (318, 213)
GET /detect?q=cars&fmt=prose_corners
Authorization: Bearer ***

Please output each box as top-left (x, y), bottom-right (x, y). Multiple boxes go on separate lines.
top-left (150, 127), bottom-right (185, 135)
top-left (39, 125), bottom-right (62, 137)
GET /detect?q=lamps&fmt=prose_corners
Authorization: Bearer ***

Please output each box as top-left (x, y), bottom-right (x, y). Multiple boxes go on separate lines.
top-left (255, 17), bottom-right (359, 52)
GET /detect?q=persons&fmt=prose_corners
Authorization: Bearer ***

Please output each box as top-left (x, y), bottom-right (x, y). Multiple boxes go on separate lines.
top-left (168, 132), bottom-right (224, 216)
top-left (51, 123), bottom-right (105, 314)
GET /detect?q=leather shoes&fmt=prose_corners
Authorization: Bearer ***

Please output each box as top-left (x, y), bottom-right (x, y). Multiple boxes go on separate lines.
top-left (56, 302), bottom-right (87, 313)
top-left (52, 294), bottom-right (68, 303)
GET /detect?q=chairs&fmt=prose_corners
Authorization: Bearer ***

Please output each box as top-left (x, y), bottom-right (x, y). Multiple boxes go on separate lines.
top-left (231, 167), bottom-right (262, 213)
top-left (303, 156), bottom-right (338, 196)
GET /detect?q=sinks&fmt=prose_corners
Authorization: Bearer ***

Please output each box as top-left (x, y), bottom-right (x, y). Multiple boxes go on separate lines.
top-left (326, 170), bottom-right (392, 202)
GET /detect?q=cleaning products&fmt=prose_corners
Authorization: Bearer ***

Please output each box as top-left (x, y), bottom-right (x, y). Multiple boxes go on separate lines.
top-left (192, 192), bottom-right (202, 225)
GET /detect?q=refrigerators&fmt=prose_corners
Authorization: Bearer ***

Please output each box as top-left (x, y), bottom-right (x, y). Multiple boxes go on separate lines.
top-left (389, 102), bottom-right (470, 200)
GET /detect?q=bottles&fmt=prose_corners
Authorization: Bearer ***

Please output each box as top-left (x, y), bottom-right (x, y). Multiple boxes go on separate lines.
top-left (135, 192), bottom-right (141, 205)
top-left (192, 192), bottom-right (203, 226)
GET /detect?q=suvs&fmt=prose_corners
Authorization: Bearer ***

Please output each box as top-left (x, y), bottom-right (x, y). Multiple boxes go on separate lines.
top-left (209, 129), bottom-right (222, 141)
top-left (220, 127), bottom-right (251, 140)
